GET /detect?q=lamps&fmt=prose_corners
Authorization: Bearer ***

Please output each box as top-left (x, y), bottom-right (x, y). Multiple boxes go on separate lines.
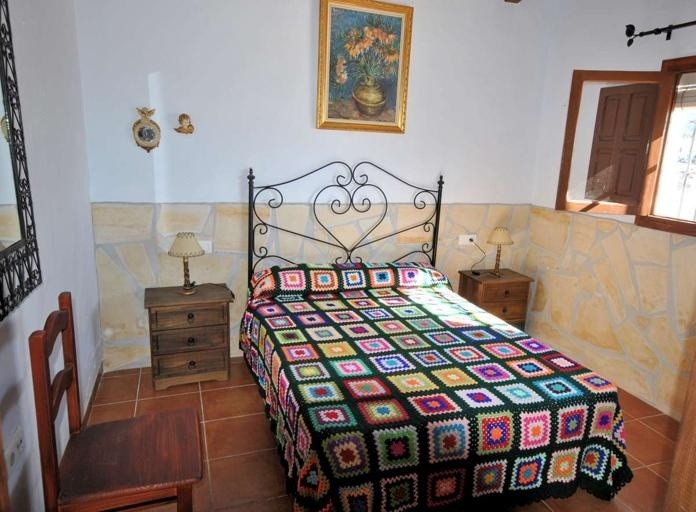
top-left (487, 227), bottom-right (514, 278)
top-left (167, 232), bottom-right (204, 296)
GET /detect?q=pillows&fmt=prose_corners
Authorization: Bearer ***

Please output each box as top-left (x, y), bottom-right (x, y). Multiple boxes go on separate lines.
top-left (248, 260), bottom-right (450, 301)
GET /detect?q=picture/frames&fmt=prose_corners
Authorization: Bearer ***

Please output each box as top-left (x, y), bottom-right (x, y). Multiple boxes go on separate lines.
top-left (315, 0), bottom-right (414, 134)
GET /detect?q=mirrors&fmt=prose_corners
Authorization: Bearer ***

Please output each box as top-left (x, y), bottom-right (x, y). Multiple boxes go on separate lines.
top-left (1, 1), bottom-right (41, 326)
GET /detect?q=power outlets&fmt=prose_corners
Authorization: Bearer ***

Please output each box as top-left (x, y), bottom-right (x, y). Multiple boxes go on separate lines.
top-left (459, 234), bottom-right (477, 245)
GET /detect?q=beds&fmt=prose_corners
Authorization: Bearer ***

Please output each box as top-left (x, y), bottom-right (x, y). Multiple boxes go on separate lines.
top-left (242, 162), bottom-right (619, 512)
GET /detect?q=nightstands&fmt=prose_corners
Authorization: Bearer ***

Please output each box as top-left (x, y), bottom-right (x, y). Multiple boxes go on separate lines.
top-left (457, 268), bottom-right (535, 333)
top-left (143, 282), bottom-right (235, 391)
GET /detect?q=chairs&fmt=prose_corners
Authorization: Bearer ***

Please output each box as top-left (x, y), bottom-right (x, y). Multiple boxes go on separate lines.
top-left (28, 290), bottom-right (204, 512)
